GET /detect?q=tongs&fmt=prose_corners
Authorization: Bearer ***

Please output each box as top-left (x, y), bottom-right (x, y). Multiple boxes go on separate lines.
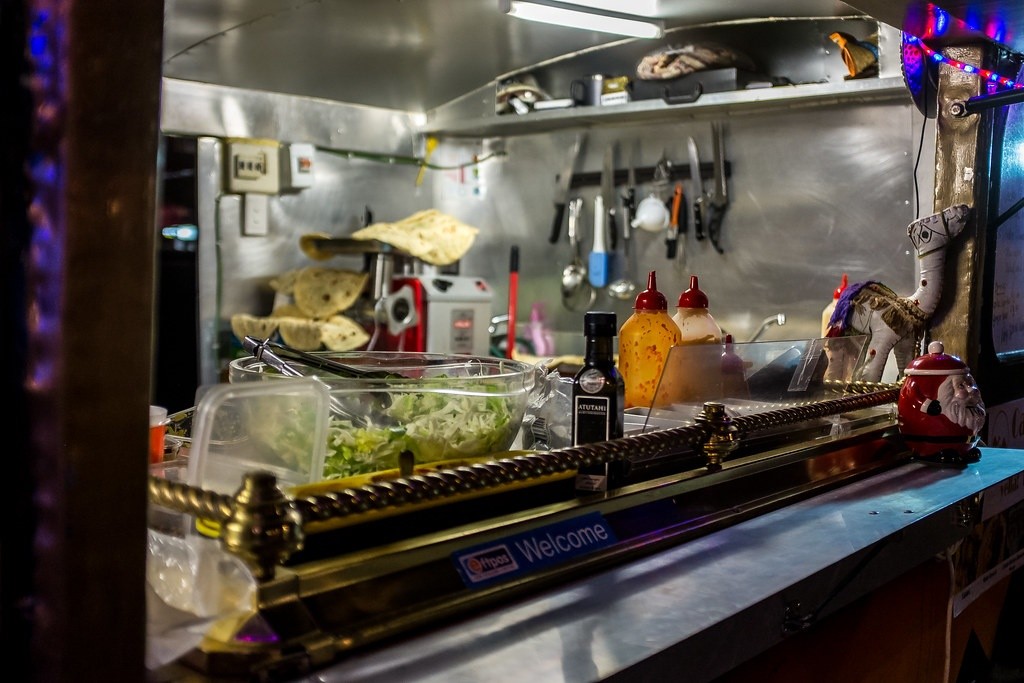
top-left (243, 334), bottom-right (418, 430)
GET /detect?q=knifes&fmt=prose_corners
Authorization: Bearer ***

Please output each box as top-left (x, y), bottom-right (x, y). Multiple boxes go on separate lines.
top-left (548, 134), bottom-right (579, 244)
top-left (602, 146), bottom-right (617, 249)
top-left (627, 161), bottom-right (637, 219)
top-left (710, 123), bottom-right (727, 255)
top-left (686, 137), bottom-right (706, 240)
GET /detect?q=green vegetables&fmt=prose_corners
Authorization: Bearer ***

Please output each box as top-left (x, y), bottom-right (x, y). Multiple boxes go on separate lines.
top-left (252, 373), bottom-right (516, 479)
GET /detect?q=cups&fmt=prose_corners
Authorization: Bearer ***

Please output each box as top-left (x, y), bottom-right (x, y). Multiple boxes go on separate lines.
top-left (590, 73), bottom-right (604, 107)
top-left (898, 342), bottom-right (985, 457)
top-left (149, 406), bottom-right (169, 461)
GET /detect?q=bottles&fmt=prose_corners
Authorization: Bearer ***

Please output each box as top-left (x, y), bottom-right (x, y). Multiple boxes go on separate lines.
top-left (673, 277), bottom-right (724, 403)
top-left (529, 304), bottom-right (552, 358)
top-left (820, 276), bottom-right (850, 382)
top-left (572, 313), bottom-right (624, 446)
top-left (618, 272), bottom-right (682, 408)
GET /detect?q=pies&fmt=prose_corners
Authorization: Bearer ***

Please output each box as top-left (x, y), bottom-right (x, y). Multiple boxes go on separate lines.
top-left (350, 208), bottom-right (478, 265)
top-left (299, 233), bottom-right (337, 261)
top-left (230, 264), bottom-right (371, 353)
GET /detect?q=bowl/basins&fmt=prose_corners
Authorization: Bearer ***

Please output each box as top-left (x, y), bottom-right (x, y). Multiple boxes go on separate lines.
top-left (165, 400), bottom-right (250, 453)
top-left (164, 436), bottom-right (183, 460)
top-left (230, 351), bottom-right (534, 478)
